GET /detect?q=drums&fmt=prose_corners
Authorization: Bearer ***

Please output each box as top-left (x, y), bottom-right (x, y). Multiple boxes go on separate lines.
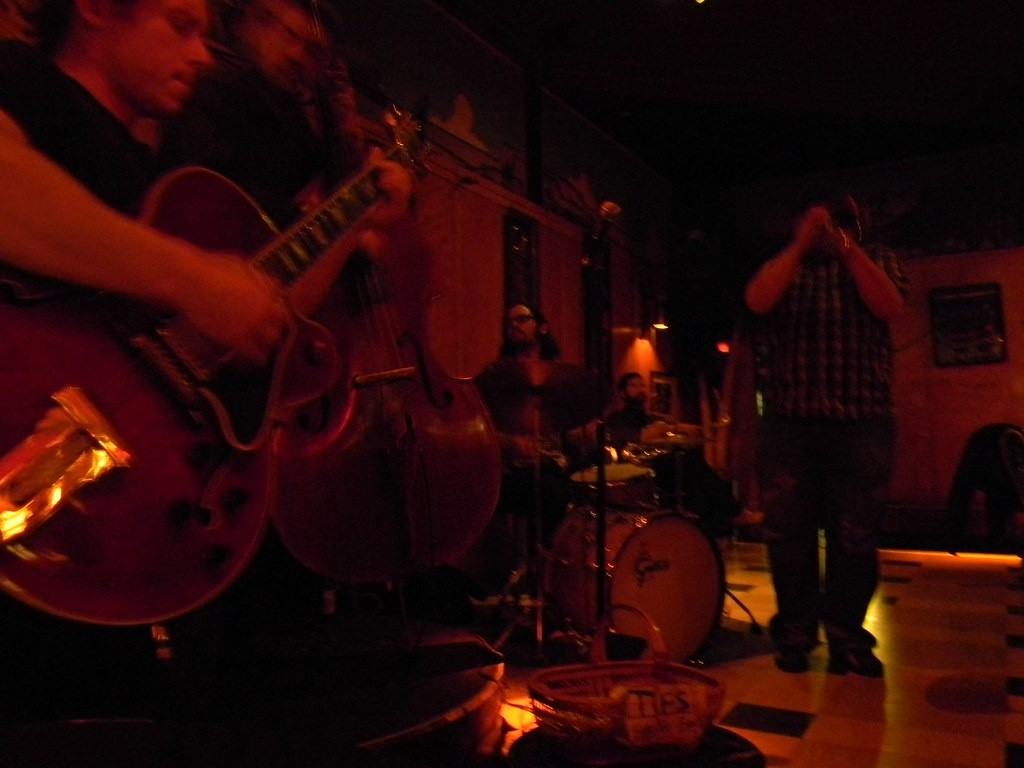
top-left (545, 502), bottom-right (726, 664)
top-left (568, 464), bottom-right (654, 501)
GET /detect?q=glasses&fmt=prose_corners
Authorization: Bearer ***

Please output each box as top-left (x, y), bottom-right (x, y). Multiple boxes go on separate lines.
top-left (503, 315), bottom-right (539, 327)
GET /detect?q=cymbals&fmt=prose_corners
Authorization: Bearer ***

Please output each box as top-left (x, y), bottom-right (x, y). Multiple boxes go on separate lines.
top-left (472, 358), bottom-right (611, 435)
top-left (639, 434), bottom-right (717, 450)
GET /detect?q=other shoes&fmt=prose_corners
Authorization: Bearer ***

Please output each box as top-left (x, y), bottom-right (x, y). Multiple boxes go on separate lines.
top-left (768, 640), bottom-right (810, 673)
top-left (829, 640), bottom-right (884, 677)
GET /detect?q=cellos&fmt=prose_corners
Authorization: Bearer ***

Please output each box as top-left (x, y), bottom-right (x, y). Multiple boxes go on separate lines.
top-left (263, 0), bottom-right (501, 642)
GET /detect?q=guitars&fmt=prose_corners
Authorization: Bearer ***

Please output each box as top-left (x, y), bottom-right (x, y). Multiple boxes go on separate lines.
top-left (0, 103), bottom-right (428, 628)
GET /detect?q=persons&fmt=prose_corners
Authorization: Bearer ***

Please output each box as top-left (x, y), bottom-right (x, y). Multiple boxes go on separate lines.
top-left (177, 1), bottom-right (363, 231)
top-left (610, 374), bottom-right (765, 531)
top-left (748, 183), bottom-right (903, 676)
top-left (484, 302), bottom-right (624, 538)
top-left (0, 1), bottom-right (300, 358)
